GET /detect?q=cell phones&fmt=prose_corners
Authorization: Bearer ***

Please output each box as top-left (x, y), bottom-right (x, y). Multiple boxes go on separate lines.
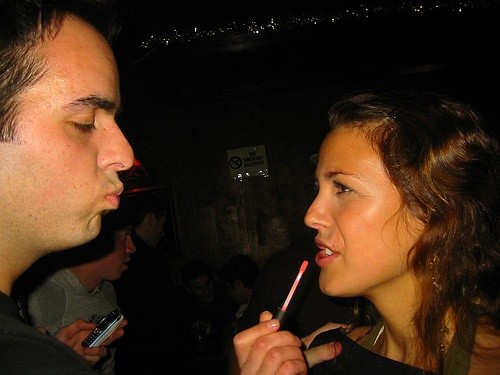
top-left (81, 310), bottom-right (124, 349)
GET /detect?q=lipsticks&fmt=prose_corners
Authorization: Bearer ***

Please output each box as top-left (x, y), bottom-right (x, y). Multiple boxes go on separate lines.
top-left (270, 261), bottom-right (310, 332)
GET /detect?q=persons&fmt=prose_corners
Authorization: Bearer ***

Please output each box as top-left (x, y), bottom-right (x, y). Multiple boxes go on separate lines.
top-left (232, 88), bottom-right (500, 375)
top-left (171, 261), bottom-right (230, 358)
top-left (215, 253), bottom-right (280, 374)
top-left (110, 191), bottom-right (174, 375)
top-left (1, 1), bottom-right (352, 375)
top-left (14, 211), bottom-right (136, 375)
